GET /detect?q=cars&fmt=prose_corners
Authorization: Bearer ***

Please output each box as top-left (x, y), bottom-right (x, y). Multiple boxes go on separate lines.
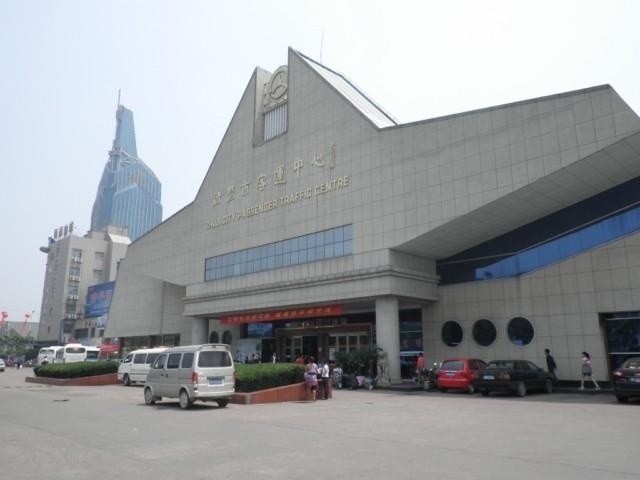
top-left (5, 359), bottom-right (37, 367)
top-left (0, 359), bottom-right (5, 372)
top-left (613, 357), bottom-right (640, 403)
top-left (475, 359), bottom-right (554, 396)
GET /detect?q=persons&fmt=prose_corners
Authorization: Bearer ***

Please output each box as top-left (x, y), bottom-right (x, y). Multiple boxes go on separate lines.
top-left (294, 352), bottom-right (343, 401)
top-left (41, 356), bottom-right (50, 365)
top-left (269, 351), bottom-right (279, 363)
top-left (416, 353), bottom-right (425, 385)
top-left (577, 352), bottom-right (600, 391)
top-left (544, 348), bottom-right (559, 387)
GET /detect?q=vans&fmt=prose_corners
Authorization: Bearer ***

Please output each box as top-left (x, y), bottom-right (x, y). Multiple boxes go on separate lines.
top-left (437, 359), bottom-right (486, 393)
top-left (117, 346), bottom-right (169, 386)
top-left (144, 343), bottom-right (236, 409)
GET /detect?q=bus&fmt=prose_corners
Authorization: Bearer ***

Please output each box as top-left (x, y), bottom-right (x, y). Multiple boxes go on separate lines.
top-left (38, 345), bottom-right (99, 365)
top-left (64, 344), bottom-right (88, 363)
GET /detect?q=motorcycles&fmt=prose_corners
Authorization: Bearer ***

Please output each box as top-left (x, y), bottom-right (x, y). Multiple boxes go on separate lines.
top-left (420, 362), bottom-right (438, 391)
